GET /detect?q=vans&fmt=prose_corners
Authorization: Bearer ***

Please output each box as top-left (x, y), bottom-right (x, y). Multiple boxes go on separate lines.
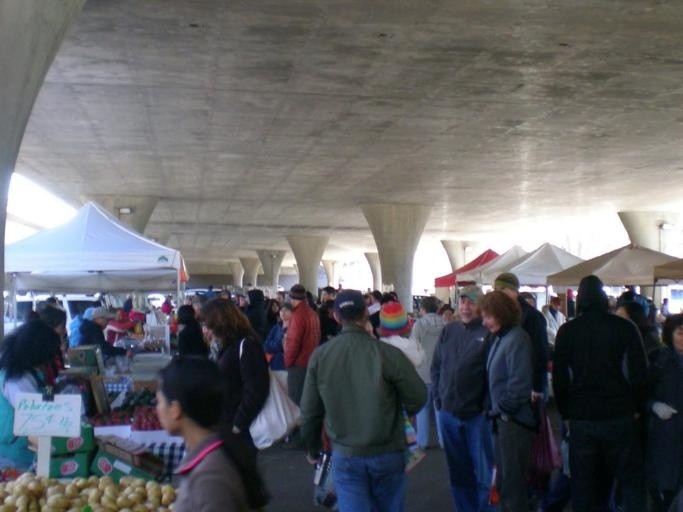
top-left (3, 293), bottom-right (104, 337)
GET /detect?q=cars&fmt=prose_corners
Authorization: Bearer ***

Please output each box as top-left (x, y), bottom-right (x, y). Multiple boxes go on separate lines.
top-left (148, 294), bottom-right (165, 308)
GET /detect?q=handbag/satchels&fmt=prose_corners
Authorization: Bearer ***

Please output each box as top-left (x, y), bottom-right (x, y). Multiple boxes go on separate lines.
top-left (528, 395), bottom-right (565, 476)
top-left (246, 364), bottom-right (303, 452)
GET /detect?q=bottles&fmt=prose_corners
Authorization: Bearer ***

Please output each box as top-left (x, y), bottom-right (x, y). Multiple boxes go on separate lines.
top-left (312, 451), bottom-right (334, 488)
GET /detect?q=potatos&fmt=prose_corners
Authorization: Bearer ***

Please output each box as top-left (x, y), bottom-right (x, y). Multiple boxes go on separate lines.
top-left (0, 471), bottom-right (176, 512)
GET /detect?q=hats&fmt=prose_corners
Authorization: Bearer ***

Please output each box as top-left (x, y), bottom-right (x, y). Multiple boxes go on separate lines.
top-left (492, 271), bottom-right (520, 292)
top-left (332, 289), bottom-right (366, 321)
top-left (457, 284), bottom-right (483, 305)
top-left (575, 275), bottom-right (609, 310)
top-left (287, 283), bottom-right (307, 302)
top-left (375, 302), bottom-right (413, 337)
top-left (90, 306), bottom-right (116, 321)
